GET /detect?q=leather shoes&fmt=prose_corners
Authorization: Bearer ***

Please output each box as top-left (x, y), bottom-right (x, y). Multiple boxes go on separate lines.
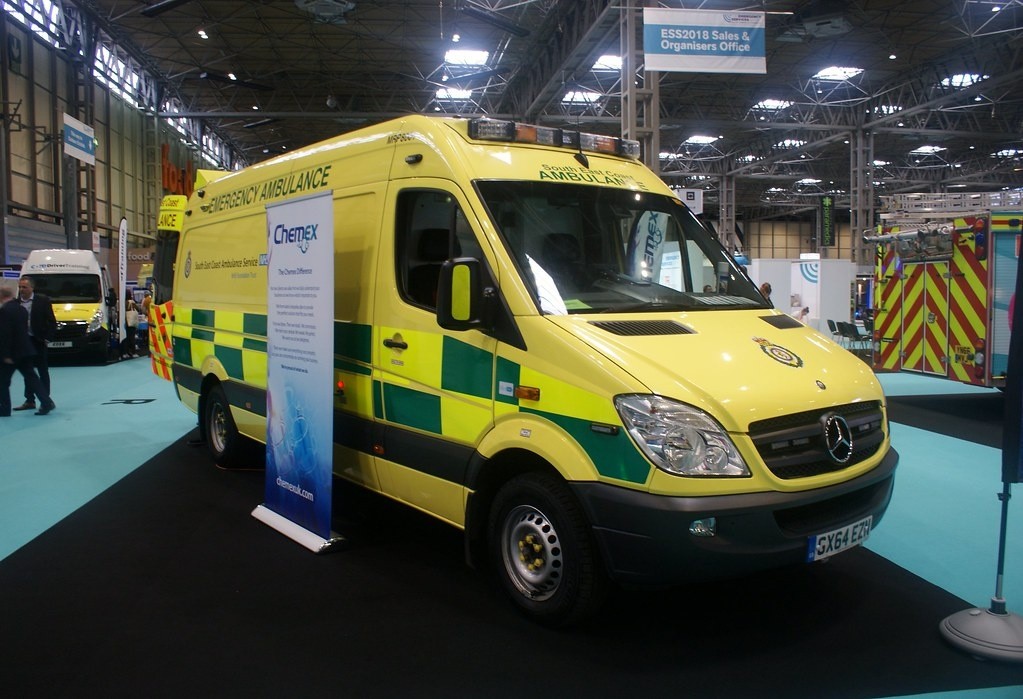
top-left (34, 401), bottom-right (55, 415)
top-left (13, 402), bottom-right (36, 410)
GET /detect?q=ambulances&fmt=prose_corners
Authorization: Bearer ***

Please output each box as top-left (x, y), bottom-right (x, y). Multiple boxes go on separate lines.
top-left (137, 264), bottom-right (154, 310)
top-left (17, 249), bottom-right (116, 364)
top-left (147, 112), bottom-right (899, 625)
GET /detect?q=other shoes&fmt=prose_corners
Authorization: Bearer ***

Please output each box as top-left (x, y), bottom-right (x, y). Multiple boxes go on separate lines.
top-left (123, 354), bottom-right (130, 358)
top-left (0, 409), bottom-right (11, 416)
top-left (132, 354), bottom-right (139, 358)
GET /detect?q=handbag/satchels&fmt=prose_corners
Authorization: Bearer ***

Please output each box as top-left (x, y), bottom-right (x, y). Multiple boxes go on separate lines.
top-left (126, 310), bottom-right (139, 328)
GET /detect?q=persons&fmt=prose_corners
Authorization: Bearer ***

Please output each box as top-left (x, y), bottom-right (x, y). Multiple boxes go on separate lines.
top-left (13, 276), bottom-right (58, 415)
top-left (0, 285), bottom-right (56, 416)
top-left (792, 306), bottom-right (809, 321)
top-left (105, 287), bottom-right (117, 341)
top-left (702, 280), bottom-right (774, 309)
top-left (121, 283), bottom-right (153, 358)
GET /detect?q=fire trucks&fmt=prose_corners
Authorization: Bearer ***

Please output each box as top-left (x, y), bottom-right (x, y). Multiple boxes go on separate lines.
top-left (860, 212), bottom-right (1023, 395)
